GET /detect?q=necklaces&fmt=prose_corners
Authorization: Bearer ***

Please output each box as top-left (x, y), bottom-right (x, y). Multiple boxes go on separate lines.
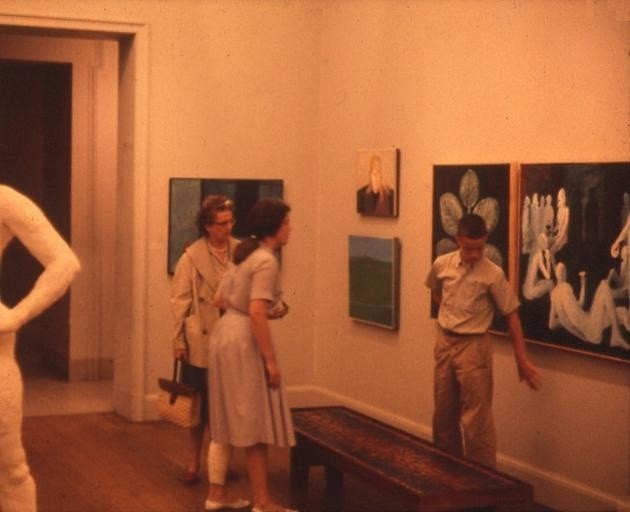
top-left (209, 241), bottom-right (227, 254)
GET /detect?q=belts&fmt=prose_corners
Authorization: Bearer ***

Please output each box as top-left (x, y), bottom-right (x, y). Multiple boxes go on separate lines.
top-left (437, 323), bottom-right (486, 336)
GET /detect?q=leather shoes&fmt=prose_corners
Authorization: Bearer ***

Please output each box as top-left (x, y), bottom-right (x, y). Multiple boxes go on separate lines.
top-left (182, 465), bottom-right (198, 483)
top-left (205, 498), bottom-right (299, 512)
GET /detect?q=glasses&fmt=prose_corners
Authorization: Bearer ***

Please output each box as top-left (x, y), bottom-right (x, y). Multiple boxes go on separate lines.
top-left (212, 218), bottom-right (236, 226)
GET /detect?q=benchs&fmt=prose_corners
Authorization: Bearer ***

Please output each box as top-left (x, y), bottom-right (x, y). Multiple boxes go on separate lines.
top-left (286, 406), bottom-right (534, 512)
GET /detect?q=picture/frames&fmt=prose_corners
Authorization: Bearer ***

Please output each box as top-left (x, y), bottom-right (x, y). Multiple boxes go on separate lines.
top-left (515, 161), bottom-right (630, 364)
top-left (168, 178), bottom-right (284, 276)
top-left (349, 148), bottom-right (401, 218)
top-left (348, 233), bottom-right (399, 332)
top-left (432, 163), bottom-right (511, 337)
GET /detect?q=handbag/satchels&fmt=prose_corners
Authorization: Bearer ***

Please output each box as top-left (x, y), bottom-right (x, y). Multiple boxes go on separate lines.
top-left (158, 378), bottom-right (201, 427)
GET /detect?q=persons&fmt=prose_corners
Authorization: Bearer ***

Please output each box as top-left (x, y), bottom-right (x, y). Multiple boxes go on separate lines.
top-left (520, 190), bottom-right (630, 351)
top-left (357, 154), bottom-right (394, 216)
top-left (423, 213), bottom-right (546, 470)
top-left (205, 196), bottom-right (298, 512)
top-left (1, 184), bottom-right (83, 512)
